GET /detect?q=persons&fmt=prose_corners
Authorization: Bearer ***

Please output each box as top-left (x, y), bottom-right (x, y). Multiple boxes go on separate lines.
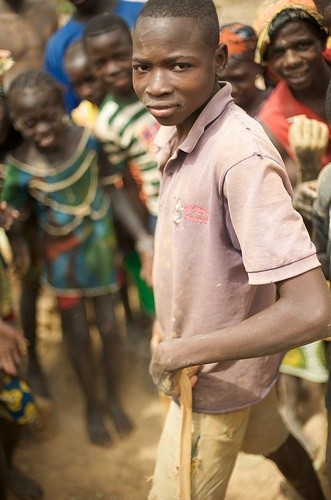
top-left (131, 0), bottom-right (331, 500)
top-left (217, 0), bottom-right (331, 500)
top-left (0, 0), bottom-right (178, 500)
